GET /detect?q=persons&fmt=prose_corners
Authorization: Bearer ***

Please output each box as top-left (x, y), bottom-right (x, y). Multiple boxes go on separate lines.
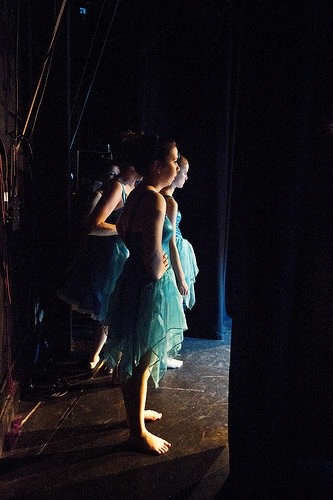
top-left (99, 129), bottom-right (189, 456)
top-left (85, 129), bottom-right (199, 375)
